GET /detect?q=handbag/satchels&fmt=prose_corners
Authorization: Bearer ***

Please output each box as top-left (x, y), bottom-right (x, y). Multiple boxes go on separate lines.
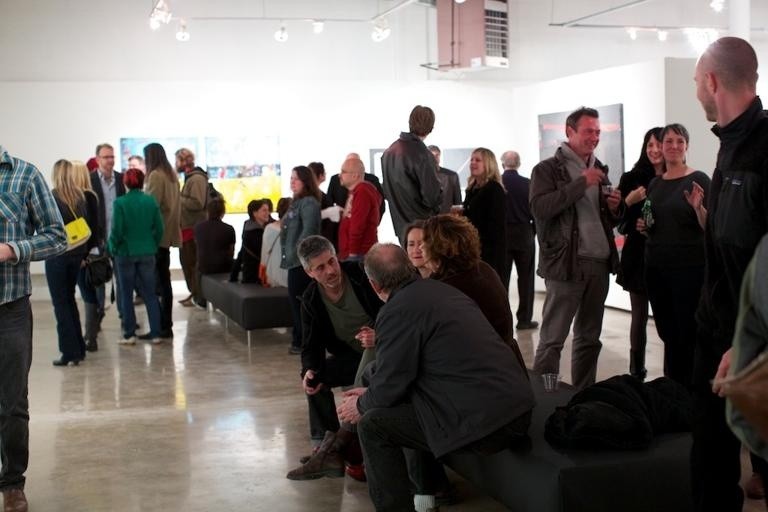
top-left (84, 254), bottom-right (113, 288)
top-left (64, 217), bottom-right (91, 251)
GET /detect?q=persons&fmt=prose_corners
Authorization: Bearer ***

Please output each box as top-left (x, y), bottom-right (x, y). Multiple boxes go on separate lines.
top-left (189, 197), bottom-right (236, 310)
top-left (615, 127), bottom-right (667, 387)
top-left (382, 105), bottom-right (539, 330)
top-left (693, 35), bottom-right (768, 345)
top-left (637, 123), bottom-right (712, 383)
top-left (43, 143), bottom-right (212, 366)
top-left (747, 451), bottom-right (766, 500)
top-left (1, 141), bottom-right (67, 511)
top-left (238, 153), bottom-right (385, 353)
top-left (287, 215), bottom-right (541, 510)
top-left (528, 104), bottom-right (621, 390)
top-left (712, 233), bottom-right (768, 461)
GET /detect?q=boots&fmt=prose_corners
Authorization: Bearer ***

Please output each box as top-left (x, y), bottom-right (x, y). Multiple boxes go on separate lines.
top-left (287, 430), bottom-right (355, 481)
top-left (630, 349), bottom-right (645, 382)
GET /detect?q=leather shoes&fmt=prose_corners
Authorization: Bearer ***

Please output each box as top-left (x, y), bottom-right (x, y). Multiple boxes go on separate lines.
top-left (52, 292), bottom-right (206, 367)
top-left (346, 463), bottom-right (365, 481)
top-left (516, 320), bottom-right (538, 329)
top-left (300, 446), bottom-right (320, 464)
top-left (3, 485), bottom-right (28, 512)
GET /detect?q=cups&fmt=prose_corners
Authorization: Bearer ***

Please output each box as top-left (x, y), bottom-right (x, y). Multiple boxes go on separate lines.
top-left (602, 185), bottom-right (616, 198)
top-left (541, 373), bottom-right (562, 393)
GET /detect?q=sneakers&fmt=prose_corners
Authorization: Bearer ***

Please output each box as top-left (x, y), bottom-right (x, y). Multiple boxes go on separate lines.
top-left (747, 471), bottom-right (765, 500)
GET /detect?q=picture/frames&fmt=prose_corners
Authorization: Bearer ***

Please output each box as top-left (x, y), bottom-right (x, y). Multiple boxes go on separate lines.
top-left (369, 148), bottom-right (387, 189)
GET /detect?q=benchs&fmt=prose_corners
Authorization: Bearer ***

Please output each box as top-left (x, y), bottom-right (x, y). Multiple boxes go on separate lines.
top-left (202, 272), bottom-right (296, 345)
top-left (401, 369), bottom-right (746, 512)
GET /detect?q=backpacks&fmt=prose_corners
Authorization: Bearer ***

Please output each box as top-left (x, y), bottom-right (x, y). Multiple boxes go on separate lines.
top-left (208, 181), bottom-right (222, 204)
